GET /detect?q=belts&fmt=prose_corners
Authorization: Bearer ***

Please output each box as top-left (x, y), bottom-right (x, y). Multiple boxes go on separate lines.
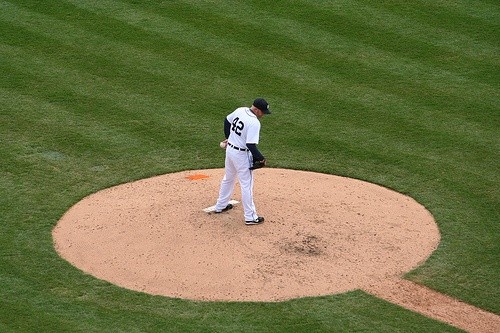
top-left (228, 142), bottom-right (249, 150)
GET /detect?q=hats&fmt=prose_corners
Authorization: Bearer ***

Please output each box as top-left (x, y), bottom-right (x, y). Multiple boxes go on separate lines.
top-left (253, 97), bottom-right (272, 114)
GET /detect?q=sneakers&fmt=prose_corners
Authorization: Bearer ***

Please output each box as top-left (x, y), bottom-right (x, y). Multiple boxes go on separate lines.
top-left (215, 203), bottom-right (233, 213)
top-left (245, 216), bottom-right (264, 224)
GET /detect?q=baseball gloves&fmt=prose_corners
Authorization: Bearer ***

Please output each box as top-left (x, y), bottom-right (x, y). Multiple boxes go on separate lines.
top-left (248, 154), bottom-right (267, 170)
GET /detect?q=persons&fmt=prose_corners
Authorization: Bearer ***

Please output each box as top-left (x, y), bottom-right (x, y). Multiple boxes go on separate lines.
top-left (214, 98), bottom-right (272, 225)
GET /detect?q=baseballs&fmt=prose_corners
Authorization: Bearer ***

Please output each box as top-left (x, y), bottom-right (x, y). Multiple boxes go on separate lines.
top-left (220, 142), bottom-right (226, 148)
